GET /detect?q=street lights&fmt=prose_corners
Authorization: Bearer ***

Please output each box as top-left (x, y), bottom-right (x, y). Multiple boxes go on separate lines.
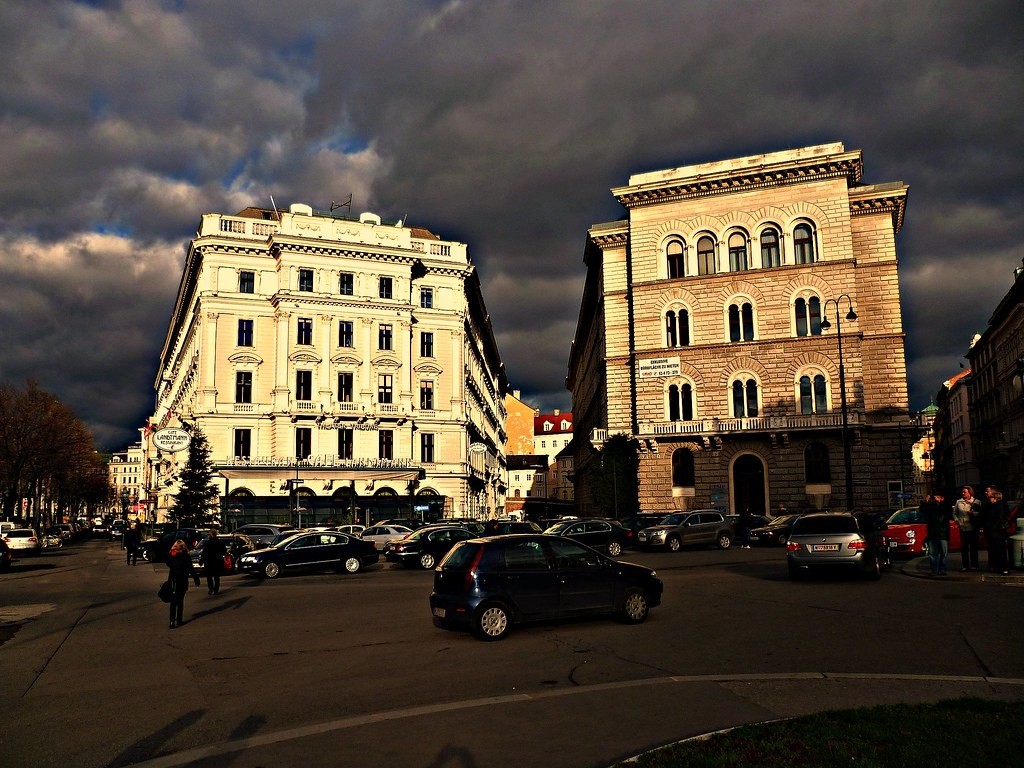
top-left (820, 292), bottom-right (858, 509)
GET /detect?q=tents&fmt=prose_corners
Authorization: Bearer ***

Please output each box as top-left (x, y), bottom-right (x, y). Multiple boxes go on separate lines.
top-left (210, 466), bottom-right (426, 524)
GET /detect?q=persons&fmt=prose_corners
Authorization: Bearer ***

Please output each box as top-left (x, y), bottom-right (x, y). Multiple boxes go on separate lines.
top-left (327, 514), bottom-right (346, 523)
top-left (230, 533), bottom-right (255, 565)
top-left (199, 529), bottom-right (227, 595)
top-left (919, 484), bottom-right (1011, 576)
top-left (739, 505), bottom-right (753, 549)
top-left (489, 522), bottom-right (505, 535)
top-left (165, 539), bottom-right (201, 628)
top-left (124, 523), bottom-right (141, 566)
top-left (776, 504), bottom-right (786, 517)
top-left (304, 537), bottom-right (314, 548)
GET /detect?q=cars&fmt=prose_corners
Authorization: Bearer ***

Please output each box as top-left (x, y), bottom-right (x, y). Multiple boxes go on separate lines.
top-left (880, 507), bottom-right (984, 555)
top-left (93, 520), bottom-right (130, 540)
top-left (130, 528), bottom-right (217, 562)
top-left (234, 530), bottom-right (379, 577)
top-left (184, 534), bottom-right (255, 577)
top-left (5, 529), bottom-right (40, 557)
top-left (637, 508), bottom-right (735, 550)
top-left (539, 519), bottom-right (635, 557)
top-left (430, 530), bottom-right (668, 643)
top-left (0, 521), bottom-right (22, 572)
top-left (785, 514), bottom-right (884, 581)
top-left (228, 518), bottom-right (537, 548)
top-left (748, 513), bottom-right (807, 544)
top-left (383, 526), bottom-right (477, 570)
top-left (39, 517), bottom-right (92, 548)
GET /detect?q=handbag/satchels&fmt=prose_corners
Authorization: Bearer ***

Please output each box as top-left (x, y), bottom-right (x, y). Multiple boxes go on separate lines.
top-left (158, 579), bottom-right (178, 603)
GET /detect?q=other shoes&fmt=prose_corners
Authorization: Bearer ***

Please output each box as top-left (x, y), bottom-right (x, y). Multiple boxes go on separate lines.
top-left (170, 622), bottom-right (175, 628)
top-left (177, 621), bottom-right (183, 625)
